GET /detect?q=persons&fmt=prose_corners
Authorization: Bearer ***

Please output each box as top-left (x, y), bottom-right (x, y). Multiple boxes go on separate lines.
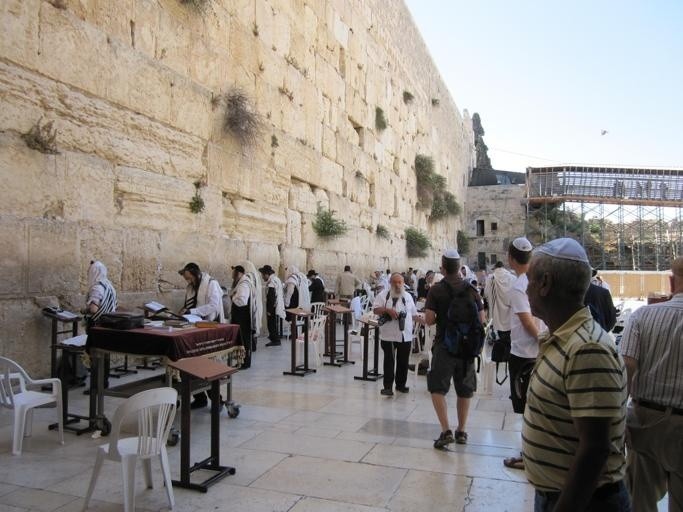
top-left (373, 272), bottom-right (416, 396)
top-left (415, 246), bottom-right (487, 448)
top-left (584, 267), bottom-right (617, 334)
top-left (620, 256), bottom-right (682, 512)
top-left (282, 266), bottom-right (310, 339)
top-left (229, 265), bottom-right (252, 372)
top-left (306, 270), bottom-right (325, 319)
top-left (334, 266), bottom-right (360, 325)
top-left (176, 263), bottom-right (224, 416)
top-left (503, 236), bottom-right (542, 469)
top-left (81, 260), bottom-right (116, 395)
top-left (484, 261), bottom-right (515, 345)
top-left (259, 265), bottom-right (285, 346)
top-left (525, 238), bottom-right (629, 512)
top-left (609, 177), bottom-right (669, 200)
top-left (370, 266), bottom-right (491, 309)
top-left (239, 259), bottom-right (263, 351)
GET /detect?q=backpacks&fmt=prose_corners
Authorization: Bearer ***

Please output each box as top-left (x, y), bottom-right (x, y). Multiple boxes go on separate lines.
top-left (440, 279), bottom-right (486, 359)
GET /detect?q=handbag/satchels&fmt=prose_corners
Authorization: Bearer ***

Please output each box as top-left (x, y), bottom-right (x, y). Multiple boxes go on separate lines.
top-left (492, 339), bottom-right (511, 362)
top-left (515, 360), bottom-right (536, 400)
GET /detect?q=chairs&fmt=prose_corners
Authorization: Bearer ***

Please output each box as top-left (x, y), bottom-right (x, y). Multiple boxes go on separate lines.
top-left (0, 356), bottom-right (67, 455)
top-left (296, 300), bottom-right (327, 367)
top-left (81, 387), bottom-right (178, 511)
top-left (405, 318), bottom-right (441, 399)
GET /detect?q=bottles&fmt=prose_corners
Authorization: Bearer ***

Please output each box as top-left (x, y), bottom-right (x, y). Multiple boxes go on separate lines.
top-left (410, 316), bottom-right (422, 335)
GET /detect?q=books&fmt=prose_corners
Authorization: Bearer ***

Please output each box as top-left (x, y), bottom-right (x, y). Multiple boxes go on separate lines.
top-left (145, 301), bottom-right (167, 313)
top-left (153, 316), bottom-right (217, 330)
top-left (58, 310), bottom-right (78, 320)
top-left (59, 335), bottom-right (88, 348)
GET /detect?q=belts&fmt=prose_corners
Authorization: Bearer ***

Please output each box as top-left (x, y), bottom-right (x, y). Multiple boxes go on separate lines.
top-left (633, 398), bottom-right (682, 415)
top-left (537, 484), bottom-right (618, 498)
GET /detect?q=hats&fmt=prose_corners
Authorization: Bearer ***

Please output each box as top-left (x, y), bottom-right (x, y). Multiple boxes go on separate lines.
top-left (442, 237), bottom-right (589, 261)
top-left (179, 258), bottom-right (317, 278)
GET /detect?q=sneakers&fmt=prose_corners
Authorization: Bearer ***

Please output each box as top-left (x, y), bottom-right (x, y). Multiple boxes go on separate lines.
top-left (381, 386), bottom-right (525, 469)
top-left (191, 335), bottom-right (297, 410)
top-left (83, 389), bottom-right (89, 394)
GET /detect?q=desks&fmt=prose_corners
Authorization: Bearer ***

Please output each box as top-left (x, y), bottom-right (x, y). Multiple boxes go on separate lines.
top-left (85, 317), bottom-right (241, 441)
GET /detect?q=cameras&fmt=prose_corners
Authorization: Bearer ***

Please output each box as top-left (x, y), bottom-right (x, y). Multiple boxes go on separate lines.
top-left (399, 310), bottom-right (406, 331)
top-left (377, 314), bottom-right (390, 326)
